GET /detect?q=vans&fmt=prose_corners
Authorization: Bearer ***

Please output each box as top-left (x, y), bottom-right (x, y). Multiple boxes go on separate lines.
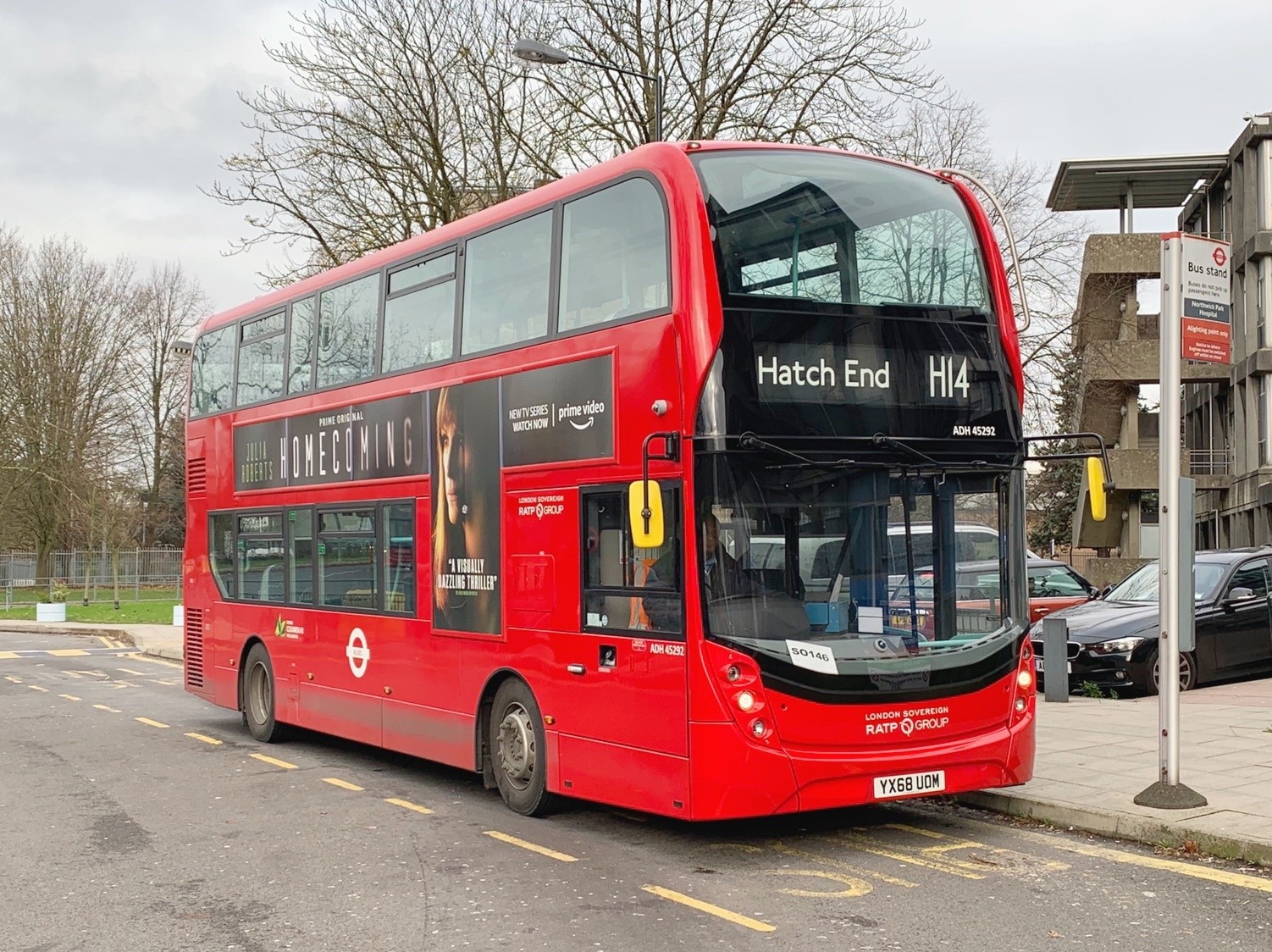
top-left (726, 519), bottom-right (1040, 635)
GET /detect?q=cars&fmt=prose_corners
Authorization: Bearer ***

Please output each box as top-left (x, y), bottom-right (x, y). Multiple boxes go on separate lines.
top-left (890, 558), bottom-right (1103, 651)
top-left (1027, 542), bottom-right (1272, 698)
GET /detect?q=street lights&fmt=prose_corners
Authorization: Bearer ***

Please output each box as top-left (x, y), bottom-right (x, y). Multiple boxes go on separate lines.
top-left (511, 39), bottom-right (665, 145)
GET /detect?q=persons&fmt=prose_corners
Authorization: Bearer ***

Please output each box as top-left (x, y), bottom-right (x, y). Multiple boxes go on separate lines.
top-left (429, 381), bottom-right (500, 635)
top-left (643, 511), bottom-right (793, 632)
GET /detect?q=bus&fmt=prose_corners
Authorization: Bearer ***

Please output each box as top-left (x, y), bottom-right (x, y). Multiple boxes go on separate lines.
top-left (182, 136), bottom-right (1118, 822)
